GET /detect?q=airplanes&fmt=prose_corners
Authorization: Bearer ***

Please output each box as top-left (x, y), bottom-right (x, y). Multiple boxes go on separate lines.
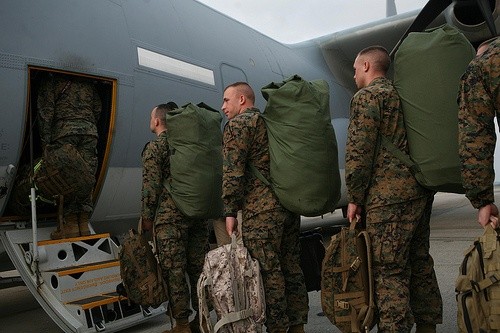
top-left (0, 0), bottom-right (500, 333)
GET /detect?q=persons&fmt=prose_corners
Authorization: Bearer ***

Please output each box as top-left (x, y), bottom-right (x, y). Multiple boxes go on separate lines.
top-left (221, 82), bottom-right (310, 333)
top-left (141, 102), bottom-right (209, 333)
top-left (344, 45), bottom-right (444, 333)
top-left (35, 72), bottom-right (102, 239)
top-left (458, 35), bottom-right (500, 229)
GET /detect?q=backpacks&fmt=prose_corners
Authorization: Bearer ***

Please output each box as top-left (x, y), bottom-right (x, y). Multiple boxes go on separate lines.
top-left (318, 217), bottom-right (378, 333)
top-left (453, 221), bottom-right (500, 333)
top-left (244, 73), bottom-right (341, 216)
top-left (159, 101), bottom-right (224, 222)
top-left (376, 24), bottom-right (477, 196)
top-left (194, 230), bottom-right (266, 333)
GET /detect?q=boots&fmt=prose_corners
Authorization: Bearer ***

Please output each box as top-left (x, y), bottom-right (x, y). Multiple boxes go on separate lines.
top-left (51, 212), bottom-right (91, 240)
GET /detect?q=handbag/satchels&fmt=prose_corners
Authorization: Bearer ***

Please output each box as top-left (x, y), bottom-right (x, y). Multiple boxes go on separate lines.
top-left (296, 231), bottom-right (327, 292)
top-left (117, 217), bottom-right (169, 309)
top-left (15, 144), bottom-right (94, 209)
